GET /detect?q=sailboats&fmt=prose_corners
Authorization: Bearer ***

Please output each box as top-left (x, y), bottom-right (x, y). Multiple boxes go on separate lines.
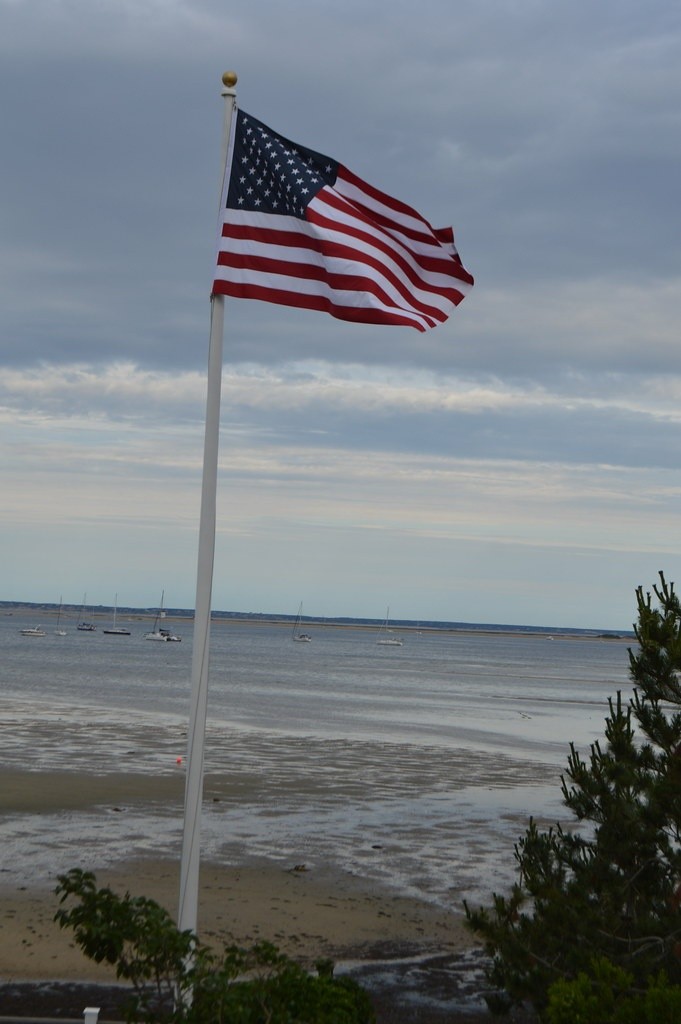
top-left (77, 592), bottom-right (98, 631)
top-left (54, 597), bottom-right (68, 636)
top-left (290, 601), bottom-right (313, 641)
top-left (103, 593), bottom-right (130, 636)
top-left (145, 591), bottom-right (182, 642)
top-left (376, 606), bottom-right (404, 645)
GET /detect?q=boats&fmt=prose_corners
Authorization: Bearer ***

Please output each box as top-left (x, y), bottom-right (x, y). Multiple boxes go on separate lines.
top-left (19, 626), bottom-right (46, 637)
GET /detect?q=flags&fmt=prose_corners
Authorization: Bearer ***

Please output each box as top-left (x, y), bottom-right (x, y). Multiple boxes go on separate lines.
top-left (160, 610), bottom-right (166, 618)
top-left (210, 107), bottom-right (474, 335)
